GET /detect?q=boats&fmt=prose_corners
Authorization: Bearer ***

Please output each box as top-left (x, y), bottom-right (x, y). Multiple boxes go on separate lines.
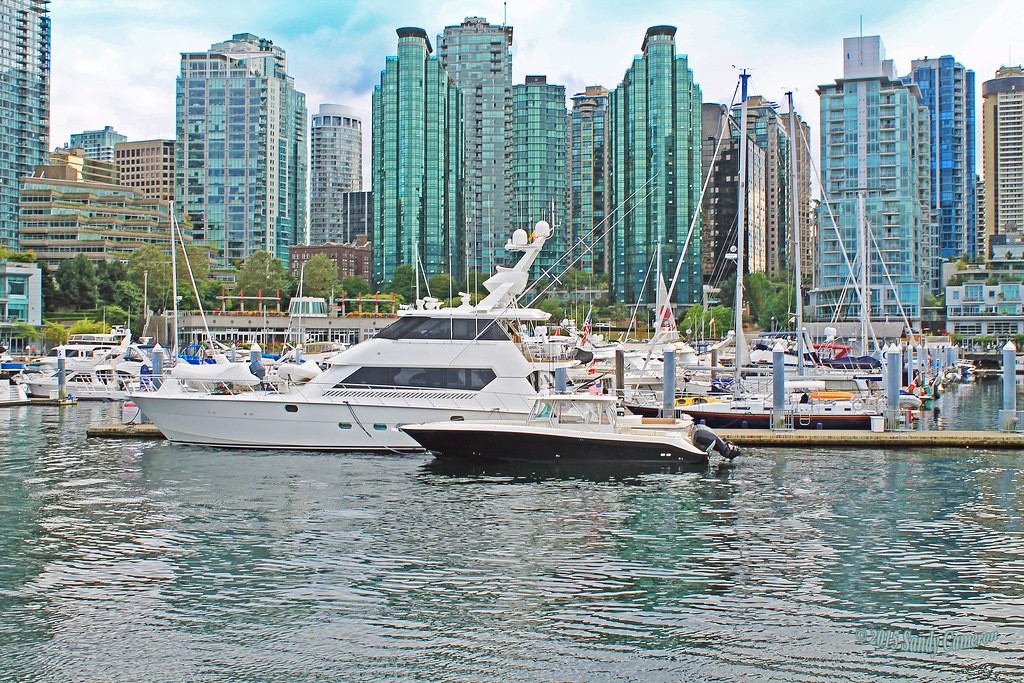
top-left (0, 62), bottom-right (977, 463)
